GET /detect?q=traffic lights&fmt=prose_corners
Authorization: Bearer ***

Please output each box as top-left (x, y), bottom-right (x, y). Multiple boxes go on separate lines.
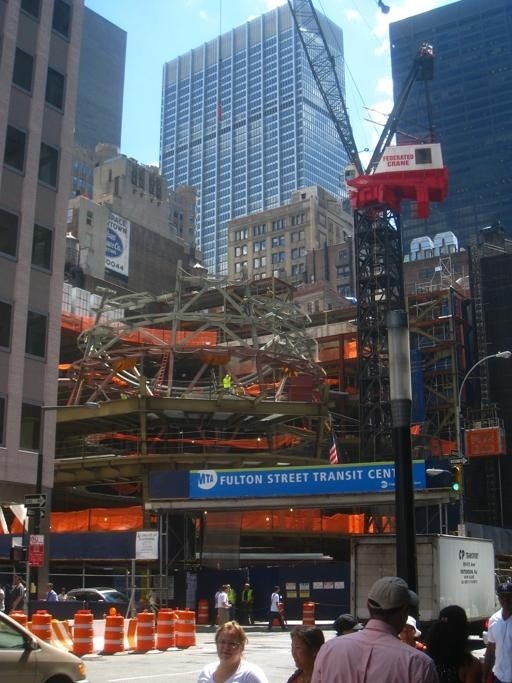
top-left (451, 464), bottom-right (462, 494)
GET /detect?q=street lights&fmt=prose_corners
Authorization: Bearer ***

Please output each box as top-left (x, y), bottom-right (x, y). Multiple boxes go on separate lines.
top-left (456, 349), bottom-right (512, 537)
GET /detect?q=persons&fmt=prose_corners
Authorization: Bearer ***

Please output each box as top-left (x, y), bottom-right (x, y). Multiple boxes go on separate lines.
top-left (222, 374), bottom-right (246, 397)
top-left (208, 582), bottom-right (255, 628)
top-left (332, 613), bottom-right (364, 636)
top-left (423, 605), bottom-right (483, 682)
top-left (310, 577), bottom-right (440, 683)
top-left (398, 616), bottom-right (427, 654)
top-left (268, 586), bottom-right (288, 629)
top-left (121, 391), bottom-right (128, 400)
top-left (197, 621), bottom-right (268, 683)
top-left (40, 583), bottom-right (59, 601)
top-left (0, 575), bottom-right (26, 615)
top-left (287, 624), bottom-right (324, 683)
top-left (480, 582), bottom-right (512, 683)
top-left (149, 588), bottom-right (156, 607)
top-left (58, 586), bottom-right (67, 601)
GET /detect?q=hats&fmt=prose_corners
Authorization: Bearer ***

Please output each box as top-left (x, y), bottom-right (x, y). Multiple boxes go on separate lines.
top-left (334, 614), bottom-right (365, 631)
top-left (497, 583), bottom-right (512, 594)
top-left (398, 615), bottom-right (421, 638)
top-left (367, 575), bottom-right (418, 610)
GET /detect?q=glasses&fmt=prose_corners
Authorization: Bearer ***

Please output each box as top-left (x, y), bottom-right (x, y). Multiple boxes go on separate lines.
top-left (218, 639), bottom-right (241, 648)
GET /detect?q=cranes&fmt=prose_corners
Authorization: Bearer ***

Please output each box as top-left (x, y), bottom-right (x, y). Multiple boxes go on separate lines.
top-left (285, 0), bottom-right (449, 535)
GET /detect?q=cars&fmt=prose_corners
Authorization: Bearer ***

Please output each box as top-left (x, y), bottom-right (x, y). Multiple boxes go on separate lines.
top-left (0, 611), bottom-right (87, 682)
top-left (66, 586), bottom-right (142, 618)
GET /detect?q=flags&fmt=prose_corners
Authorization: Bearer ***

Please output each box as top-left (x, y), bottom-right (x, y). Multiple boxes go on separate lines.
top-left (328, 426), bottom-right (339, 465)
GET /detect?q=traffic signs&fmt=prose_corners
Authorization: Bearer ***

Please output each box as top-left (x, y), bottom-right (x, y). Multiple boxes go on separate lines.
top-left (26, 507), bottom-right (45, 518)
top-left (449, 455), bottom-right (470, 465)
top-left (24, 493), bottom-right (47, 508)
